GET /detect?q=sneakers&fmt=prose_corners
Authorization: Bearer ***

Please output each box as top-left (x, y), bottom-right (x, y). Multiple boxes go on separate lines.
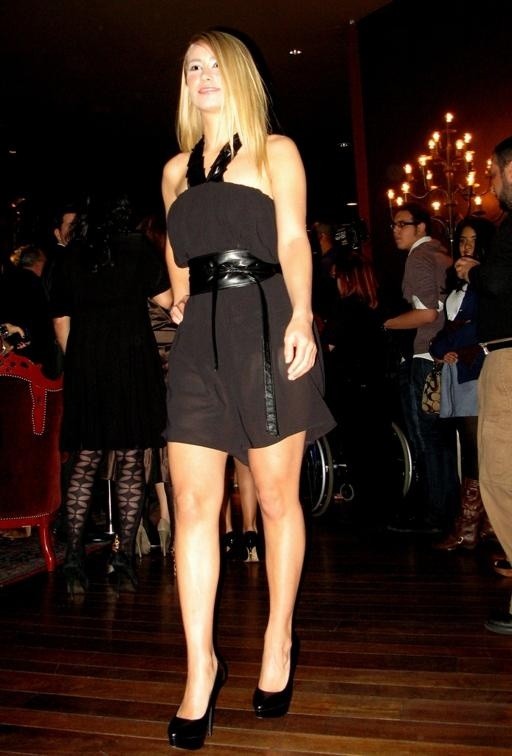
top-left (384, 508), bottom-right (443, 535)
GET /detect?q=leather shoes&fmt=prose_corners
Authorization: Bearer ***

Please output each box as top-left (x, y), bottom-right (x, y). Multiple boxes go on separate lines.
top-left (53, 526), bottom-right (114, 549)
top-left (482, 610), bottom-right (512, 637)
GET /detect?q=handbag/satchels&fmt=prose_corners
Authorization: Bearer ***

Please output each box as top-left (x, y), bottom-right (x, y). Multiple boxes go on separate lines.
top-left (421, 361), bottom-right (442, 417)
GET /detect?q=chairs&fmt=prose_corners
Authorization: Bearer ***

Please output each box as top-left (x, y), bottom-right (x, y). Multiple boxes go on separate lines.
top-left (0, 349), bottom-right (67, 576)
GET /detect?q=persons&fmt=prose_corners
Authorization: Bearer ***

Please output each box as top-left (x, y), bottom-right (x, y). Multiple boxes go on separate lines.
top-left (160, 31), bottom-right (335, 753)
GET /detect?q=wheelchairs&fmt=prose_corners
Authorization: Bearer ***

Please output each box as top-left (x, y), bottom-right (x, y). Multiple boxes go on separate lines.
top-left (301, 417), bottom-right (413, 520)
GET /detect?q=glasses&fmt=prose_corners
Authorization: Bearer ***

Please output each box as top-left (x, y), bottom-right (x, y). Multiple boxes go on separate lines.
top-left (390, 221), bottom-right (416, 229)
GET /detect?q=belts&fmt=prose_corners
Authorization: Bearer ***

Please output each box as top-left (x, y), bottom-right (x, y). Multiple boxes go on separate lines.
top-left (482, 340), bottom-right (512, 356)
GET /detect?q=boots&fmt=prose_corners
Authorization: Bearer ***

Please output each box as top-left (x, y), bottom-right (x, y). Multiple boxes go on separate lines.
top-left (433, 475), bottom-right (495, 551)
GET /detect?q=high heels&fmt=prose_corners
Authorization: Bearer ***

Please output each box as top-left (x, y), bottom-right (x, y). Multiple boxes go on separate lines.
top-left (64, 559), bottom-right (88, 599)
top-left (112, 556), bottom-right (130, 599)
top-left (252, 641), bottom-right (295, 722)
top-left (167, 657), bottom-right (229, 752)
top-left (136, 518), bottom-right (258, 559)
